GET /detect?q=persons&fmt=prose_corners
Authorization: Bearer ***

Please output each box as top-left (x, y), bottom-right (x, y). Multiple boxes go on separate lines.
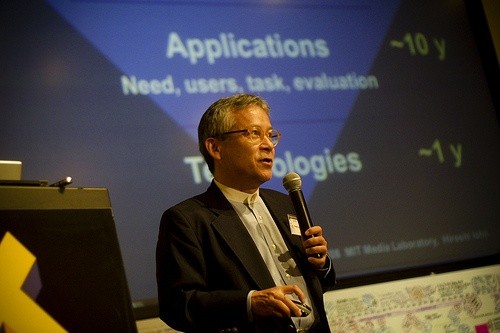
top-left (155, 92), bottom-right (337, 333)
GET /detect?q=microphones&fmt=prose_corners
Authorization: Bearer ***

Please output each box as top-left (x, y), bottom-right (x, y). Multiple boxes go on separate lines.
top-left (282, 172), bottom-right (321, 259)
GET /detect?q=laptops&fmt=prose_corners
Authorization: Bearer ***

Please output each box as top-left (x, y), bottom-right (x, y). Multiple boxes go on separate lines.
top-left (0, 161), bottom-right (22, 180)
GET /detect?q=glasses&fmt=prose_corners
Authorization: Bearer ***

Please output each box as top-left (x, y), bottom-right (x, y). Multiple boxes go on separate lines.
top-left (214, 126), bottom-right (281, 147)
